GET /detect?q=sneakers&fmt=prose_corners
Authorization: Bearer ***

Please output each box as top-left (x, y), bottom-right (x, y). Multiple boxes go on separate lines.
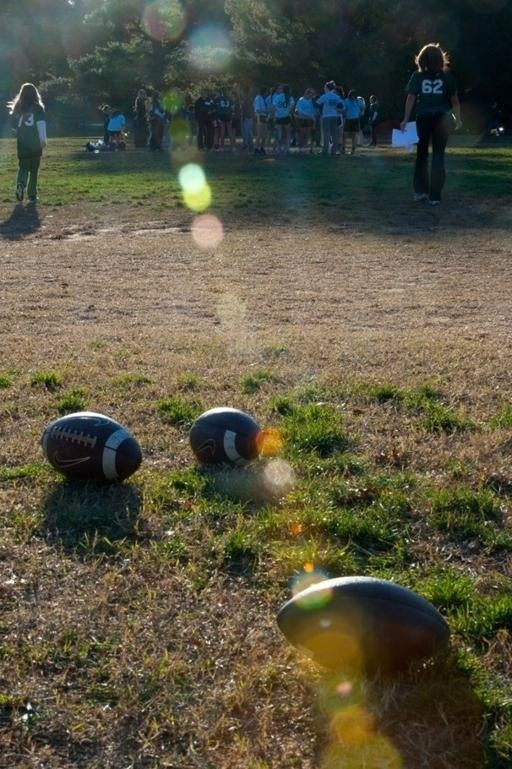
top-left (151, 144), bottom-right (194, 152)
top-left (415, 194), bottom-right (428, 202)
top-left (16, 183), bottom-right (23, 201)
top-left (429, 200), bottom-right (440, 205)
top-left (27, 198), bottom-right (38, 203)
top-left (200, 146), bottom-right (357, 155)
top-left (360, 140), bottom-right (376, 146)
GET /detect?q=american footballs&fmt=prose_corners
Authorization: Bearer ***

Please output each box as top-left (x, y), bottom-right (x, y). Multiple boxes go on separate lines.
top-left (277, 575), bottom-right (450, 673)
top-left (189, 408), bottom-right (264, 466)
top-left (40, 411), bottom-right (141, 485)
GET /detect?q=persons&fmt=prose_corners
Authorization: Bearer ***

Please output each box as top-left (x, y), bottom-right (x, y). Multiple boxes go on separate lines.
top-left (102, 80), bottom-right (379, 158)
top-left (401, 44), bottom-right (462, 206)
top-left (487, 101), bottom-right (502, 136)
top-left (8, 84), bottom-right (47, 201)
